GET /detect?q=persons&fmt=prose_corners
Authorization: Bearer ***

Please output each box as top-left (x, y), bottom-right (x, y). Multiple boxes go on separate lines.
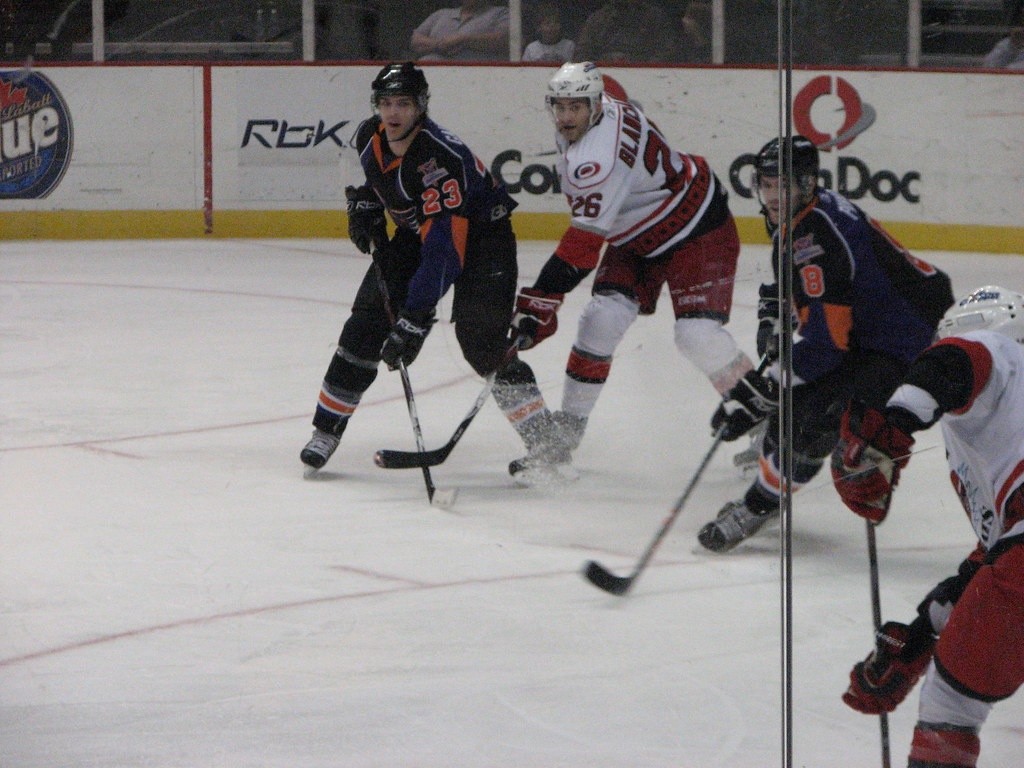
top-left (300, 63), bottom-right (573, 475)
top-left (832, 284), bottom-right (1024, 768)
top-left (410, 0), bottom-right (712, 61)
top-left (506, 60), bottom-right (769, 467)
top-left (698, 136), bottom-right (954, 553)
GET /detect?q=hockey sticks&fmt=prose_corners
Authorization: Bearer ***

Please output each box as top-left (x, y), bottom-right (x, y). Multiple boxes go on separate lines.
top-left (583, 353), bottom-right (773, 598)
top-left (373, 325), bottom-right (532, 471)
top-left (864, 516), bottom-right (893, 767)
top-left (367, 240), bottom-right (458, 513)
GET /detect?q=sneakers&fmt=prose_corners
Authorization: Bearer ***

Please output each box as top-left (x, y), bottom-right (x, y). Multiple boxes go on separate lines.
top-left (698, 487), bottom-right (784, 554)
top-left (509, 439), bottom-right (575, 487)
top-left (301, 431), bottom-right (342, 478)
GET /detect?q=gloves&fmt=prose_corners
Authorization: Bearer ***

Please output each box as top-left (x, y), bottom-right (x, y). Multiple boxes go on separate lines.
top-left (345, 184), bottom-right (382, 253)
top-left (711, 370), bottom-right (782, 441)
top-left (839, 621), bottom-right (936, 717)
top-left (757, 283), bottom-right (786, 363)
top-left (504, 288), bottom-right (565, 352)
top-left (830, 404), bottom-right (916, 525)
top-left (380, 308), bottom-right (439, 372)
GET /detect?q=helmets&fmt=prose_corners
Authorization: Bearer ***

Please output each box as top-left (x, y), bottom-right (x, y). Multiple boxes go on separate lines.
top-left (750, 136), bottom-right (821, 192)
top-left (930, 284), bottom-right (1024, 347)
top-left (545, 62), bottom-right (605, 127)
top-left (368, 61), bottom-right (431, 119)
